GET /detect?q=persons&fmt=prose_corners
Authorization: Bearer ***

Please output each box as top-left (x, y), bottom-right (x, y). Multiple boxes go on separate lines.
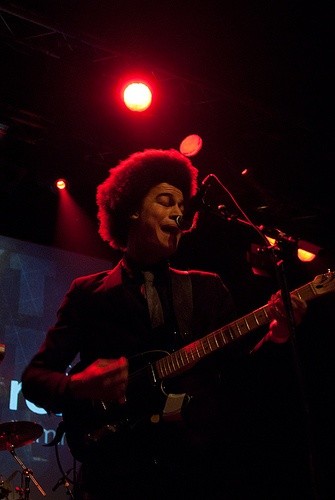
top-left (21, 147), bottom-right (307, 500)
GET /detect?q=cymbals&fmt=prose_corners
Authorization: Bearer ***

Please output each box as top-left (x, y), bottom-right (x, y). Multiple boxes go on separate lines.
top-left (0, 420), bottom-right (45, 451)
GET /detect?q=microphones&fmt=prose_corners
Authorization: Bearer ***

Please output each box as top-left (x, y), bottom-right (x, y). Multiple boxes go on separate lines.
top-left (176, 174), bottom-right (213, 230)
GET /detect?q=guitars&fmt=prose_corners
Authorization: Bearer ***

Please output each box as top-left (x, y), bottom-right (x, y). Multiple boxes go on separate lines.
top-left (62, 271), bottom-right (335, 443)
